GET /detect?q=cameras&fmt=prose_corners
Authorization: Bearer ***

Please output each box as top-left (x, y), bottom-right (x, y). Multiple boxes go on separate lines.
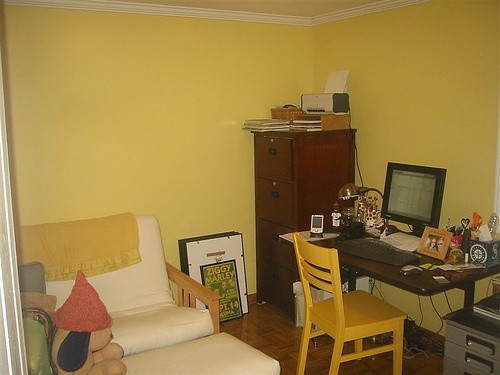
top-left (309, 215), bottom-right (325, 234)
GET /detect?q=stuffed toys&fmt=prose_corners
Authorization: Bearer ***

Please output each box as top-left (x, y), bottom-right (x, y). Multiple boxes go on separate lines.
top-left (51, 271), bottom-right (127, 375)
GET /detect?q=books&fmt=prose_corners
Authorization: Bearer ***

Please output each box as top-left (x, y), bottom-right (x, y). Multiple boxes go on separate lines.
top-left (242, 118), bottom-right (321, 131)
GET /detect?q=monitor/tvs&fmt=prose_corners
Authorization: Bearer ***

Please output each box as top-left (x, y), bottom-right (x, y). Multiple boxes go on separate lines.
top-left (381, 162), bottom-right (447, 237)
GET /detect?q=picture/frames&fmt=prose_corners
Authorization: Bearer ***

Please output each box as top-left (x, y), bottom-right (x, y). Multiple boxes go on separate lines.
top-left (417, 226), bottom-right (454, 261)
top-left (200, 259), bottom-right (243, 323)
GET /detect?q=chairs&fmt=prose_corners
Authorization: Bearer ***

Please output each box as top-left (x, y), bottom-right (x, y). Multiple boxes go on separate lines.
top-left (292, 231), bottom-right (408, 375)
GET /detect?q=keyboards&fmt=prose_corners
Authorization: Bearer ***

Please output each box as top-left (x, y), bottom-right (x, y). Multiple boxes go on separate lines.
top-left (340, 239), bottom-right (423, 266)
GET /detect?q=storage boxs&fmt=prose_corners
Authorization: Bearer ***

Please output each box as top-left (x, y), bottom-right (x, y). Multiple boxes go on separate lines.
top-left (178, 231), bottom-right (249, 315)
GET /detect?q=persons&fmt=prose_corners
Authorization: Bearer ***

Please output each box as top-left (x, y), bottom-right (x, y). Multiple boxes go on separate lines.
top-left (427, 237), bottom-right (443, 251)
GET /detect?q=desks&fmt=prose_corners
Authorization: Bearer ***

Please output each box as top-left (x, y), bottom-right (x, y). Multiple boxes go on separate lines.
top-left (278, 227), bottom-right (500, 309)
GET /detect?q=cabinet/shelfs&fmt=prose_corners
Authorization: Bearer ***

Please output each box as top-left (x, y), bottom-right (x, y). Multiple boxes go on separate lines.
top-left (442, 307), bottom-right (500, 375)
top-left (250, 128), bottom-right (358, 313)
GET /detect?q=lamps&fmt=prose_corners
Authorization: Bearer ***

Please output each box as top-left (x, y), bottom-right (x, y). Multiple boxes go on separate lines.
top-left (339, 182), bottom-right (397, 233)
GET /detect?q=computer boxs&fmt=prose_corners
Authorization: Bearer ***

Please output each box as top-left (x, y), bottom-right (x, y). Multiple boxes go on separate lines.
top-left (341, 266), bottom-right (370, 293)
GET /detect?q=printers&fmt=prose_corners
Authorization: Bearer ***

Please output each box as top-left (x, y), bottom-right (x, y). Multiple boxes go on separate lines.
top-left (301, 84), bottom-right (351, 115)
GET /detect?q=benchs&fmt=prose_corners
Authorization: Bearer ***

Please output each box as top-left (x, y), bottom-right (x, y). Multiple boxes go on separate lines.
top-left (123, 331), bottom-right (281, 375)
top-left (14, 212), bottom-right (222, 357)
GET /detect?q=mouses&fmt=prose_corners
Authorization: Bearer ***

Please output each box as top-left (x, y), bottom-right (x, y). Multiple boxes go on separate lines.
top-left (400, 265), bottom-right (423, 275)
